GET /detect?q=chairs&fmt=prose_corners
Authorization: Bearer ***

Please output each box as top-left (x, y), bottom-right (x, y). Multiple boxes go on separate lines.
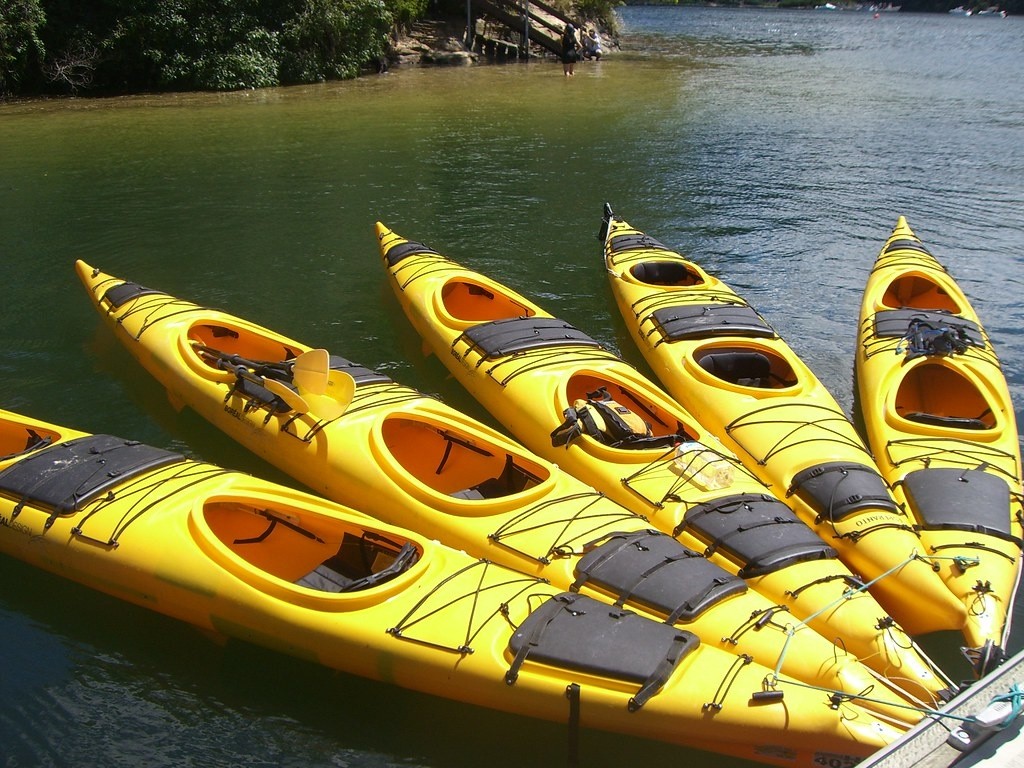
top-left (904, 412), bottom-right (986, 429)
top-left (699, 352), bottom-right (795, 389)
top-left (292, 532), bottom-right (420, 593)
top-left (608, 426), bottom-right (692, 451)
top-left (633, 262), bottom-right (700, 286)
top-left (448, 454), bottom-right (544, 501)
top-left (0, 429), bottom-right (53, 462)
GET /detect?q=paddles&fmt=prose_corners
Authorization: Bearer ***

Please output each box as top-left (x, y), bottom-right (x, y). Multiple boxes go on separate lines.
top-left (190, 337), bottom-right (330, 397)
top-left (198, 351), bottom-right (309, 415)
top-left (243, 358), bottom-right (356, 420)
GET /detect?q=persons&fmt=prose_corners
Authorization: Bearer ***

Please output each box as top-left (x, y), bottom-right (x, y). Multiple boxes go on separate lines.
top-left (580, 28), bottom-right (603, 61)
top-left (560, 23), bottom-right (583, 76)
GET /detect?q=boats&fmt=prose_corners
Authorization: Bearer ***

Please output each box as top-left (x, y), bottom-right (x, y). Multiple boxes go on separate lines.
top-left (855, 215), bottom-right (1024, 683)
top-left (373, 219), bottom-right (958, 712)
top-left (0, 409), bottom-right (908, 768)
top-left (599, 199), bottom-right (968, 645)
top-left (72, 257), bottom-right (926, 727)
